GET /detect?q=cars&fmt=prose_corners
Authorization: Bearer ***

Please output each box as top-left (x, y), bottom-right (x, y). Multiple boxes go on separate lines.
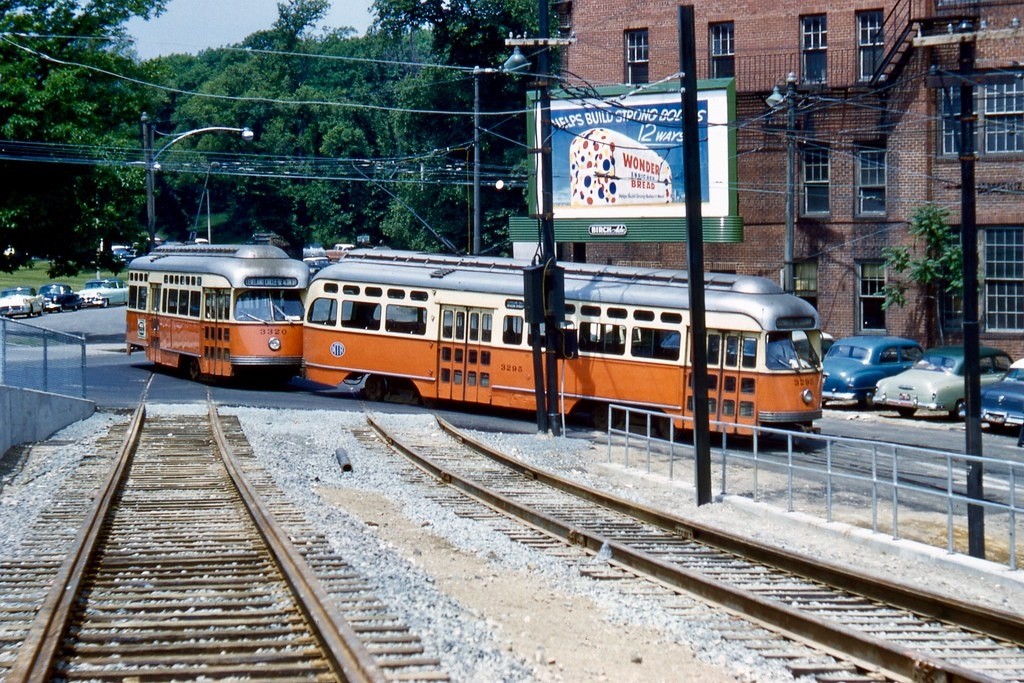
top-left (959, 357), bottom-right (1024, 427)
top-left (76, 278), bottom-right (129, 308)
top-left (872, 345), bottom-right (1013, 424)
top-left (821, 335), bottom-right (925, 411)
top-left (0, 287), bottom-right (44, 320)
top-left (38, 284), bottom-right (84, 313)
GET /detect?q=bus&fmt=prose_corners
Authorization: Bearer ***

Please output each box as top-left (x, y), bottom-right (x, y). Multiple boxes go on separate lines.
top-left (125, 170), bottom-right (313, 385)
top-left (300, 248), bottom-right (825, 437)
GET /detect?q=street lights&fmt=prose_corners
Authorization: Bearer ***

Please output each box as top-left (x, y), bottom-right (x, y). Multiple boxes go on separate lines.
top-left (473, 45), bottom-right (531, 255)
top-left (766, 73), bottom-right (797, 293)
top-left (139, 110), bottom-right (254, 252)
